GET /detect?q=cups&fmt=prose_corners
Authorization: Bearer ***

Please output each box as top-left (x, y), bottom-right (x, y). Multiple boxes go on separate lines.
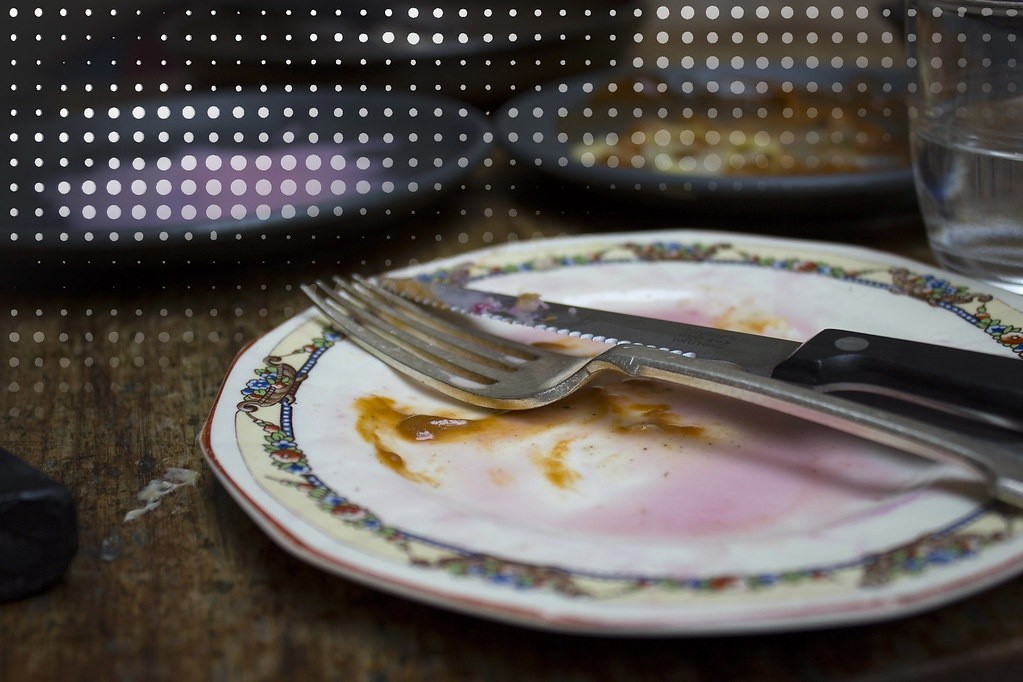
top-left (906, 0), bottom-right (1023, 293)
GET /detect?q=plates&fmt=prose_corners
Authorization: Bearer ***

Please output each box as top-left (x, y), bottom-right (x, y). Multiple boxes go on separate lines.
top-left (0, 93), bottom-right (491, 247)
top-left (501, 63), bottom-right (919, 197)
top-left (200, 226), bottom-right (1023, 636)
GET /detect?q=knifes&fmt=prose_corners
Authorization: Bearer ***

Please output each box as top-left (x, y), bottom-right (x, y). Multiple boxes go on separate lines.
top-left (380, 275), bottom-right (1023, 438)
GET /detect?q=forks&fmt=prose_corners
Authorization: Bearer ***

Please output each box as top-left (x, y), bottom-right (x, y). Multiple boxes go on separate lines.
top-left (303, 275), bottom-right (1023, 509)
